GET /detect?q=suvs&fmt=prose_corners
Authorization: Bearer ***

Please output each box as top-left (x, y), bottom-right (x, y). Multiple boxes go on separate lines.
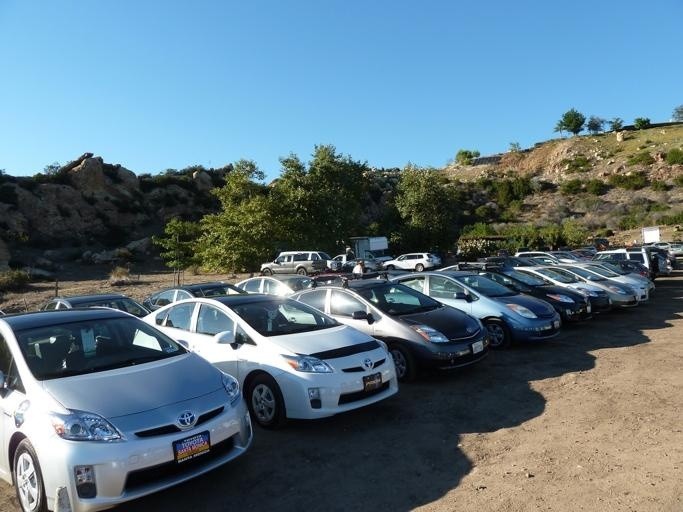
top-left (382, 252), bottom-right (434, 272)
top-left (431, 254), bottom-right (442, 267)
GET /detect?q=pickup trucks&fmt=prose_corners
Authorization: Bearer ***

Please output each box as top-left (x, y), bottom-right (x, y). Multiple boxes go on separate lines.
top-left (258, 254), bottom-right (323, 274)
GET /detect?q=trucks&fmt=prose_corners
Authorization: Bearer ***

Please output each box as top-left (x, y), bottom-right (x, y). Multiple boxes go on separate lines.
top-left (346, 236), bottom-right (393, 265)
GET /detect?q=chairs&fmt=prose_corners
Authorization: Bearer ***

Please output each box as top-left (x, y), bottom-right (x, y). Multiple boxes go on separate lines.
top-left (247, 309), bottom-right (270, 332)
top-left (34, 339), bottom-right (70, 374)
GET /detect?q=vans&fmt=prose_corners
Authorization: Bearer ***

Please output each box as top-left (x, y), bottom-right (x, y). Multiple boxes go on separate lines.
top-left (278, 250), bottom-right (330, 262)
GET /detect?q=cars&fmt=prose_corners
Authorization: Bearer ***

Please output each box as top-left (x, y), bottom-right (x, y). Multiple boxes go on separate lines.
top-left (137, 296), bottom-right (398, 425)
top-left (288, 281), bottom-right (490, 380)
top-left (144, 283), bottom-right (246, 312)
top-left (325, 254), bottom-right (375, 272)
top-left (233, 275), bottom-right (314, 296)
top-left (39, 293), bottom-right (150, 316)
top-left (312, 273), bottom-right (360, 283)
top-left (466, 246), bottom-right (683, 324)
top-left (1, 308), bottom-right (253, 511)
top-left (391, 272), bottom-right (562, 348)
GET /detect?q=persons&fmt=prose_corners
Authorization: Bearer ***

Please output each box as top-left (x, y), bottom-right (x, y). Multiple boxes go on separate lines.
top-left (352, 261), bottom-right (364, 276)
